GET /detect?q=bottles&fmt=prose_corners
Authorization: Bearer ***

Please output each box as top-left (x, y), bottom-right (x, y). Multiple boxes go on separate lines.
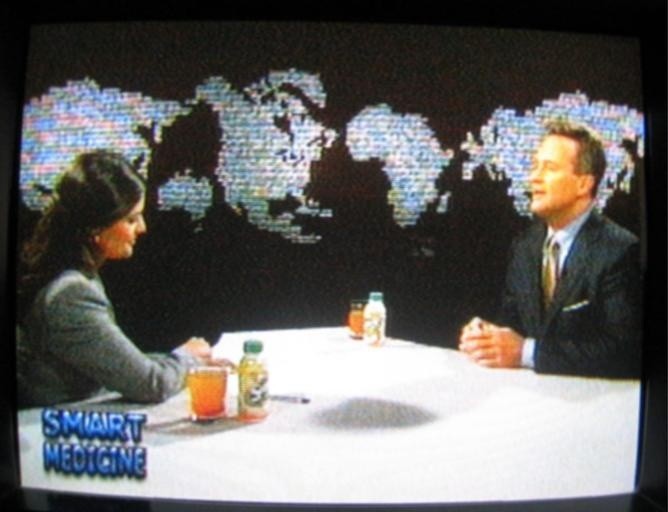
top-left (347, 299), bottom-right (370, 339)
top-left (236, 339), bottom-right (273, 421)
top-left (362, 291), bottom-right (386, 348)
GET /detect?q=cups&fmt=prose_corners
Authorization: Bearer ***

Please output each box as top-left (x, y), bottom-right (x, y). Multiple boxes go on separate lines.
top-left (185, 357), bottom-right (231, 424)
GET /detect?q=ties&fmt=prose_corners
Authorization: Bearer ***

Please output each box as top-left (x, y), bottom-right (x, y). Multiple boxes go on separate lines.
top-left (542, 236), bottom-right (560, 312)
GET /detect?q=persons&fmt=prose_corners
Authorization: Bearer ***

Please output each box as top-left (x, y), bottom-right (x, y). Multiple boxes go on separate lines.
top-left (459, 119), bottom-right (642, 380)
top-left (16, 149), bottom-right (239, 413)
